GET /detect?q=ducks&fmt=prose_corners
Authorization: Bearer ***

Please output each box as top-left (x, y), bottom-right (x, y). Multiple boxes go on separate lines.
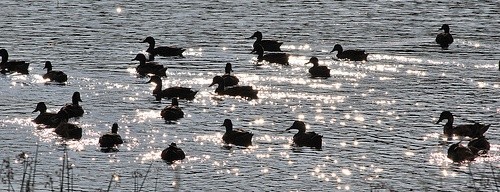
top-left (305, 57), bottom-right (331, 78)
top-left (131, 53), bottom-right (167, 78)
top-left (446, 142), bottom-right (475, 165)
top-left (435, 23), bottom-right (453, 45)
top-left (161, 97), bottom-right (184, 122)
top-left (60, 91), bottom-right (84, 117)
top-left (43, 60), bottom-right (68, 83)
top-left (436, 111), bottom-right (490, 137)
top-left (32, 102), bottom-right (58, 125)
top-left (252, 43), bottom-right (290, 66)
top-left (249, 31), bottom-right (283, 51)
top-left (161, 142), bottom-right (185, 161)
top-left (285, 120), bottom-right (323, 148)
top-left (52, 109), bottom-right (82, 139)
top-left (219, 118), bottom-right (254, 147)
top-left (208, 76), bottom-right (258, 99)
top-left (0, 48), bottom-right (30, 71)
top-left (467, 138), bottom-right (491, 156)
top-left (330, 44), bottom-right (369, 62)
top-left (138, 35), bottom-right (186, 56)
top-left (98, 121), bottom-right (124, 151)
top-left (145, 76), bottom-right (198, 100)
top-left (222, 62), bottom-right (239, 86)
